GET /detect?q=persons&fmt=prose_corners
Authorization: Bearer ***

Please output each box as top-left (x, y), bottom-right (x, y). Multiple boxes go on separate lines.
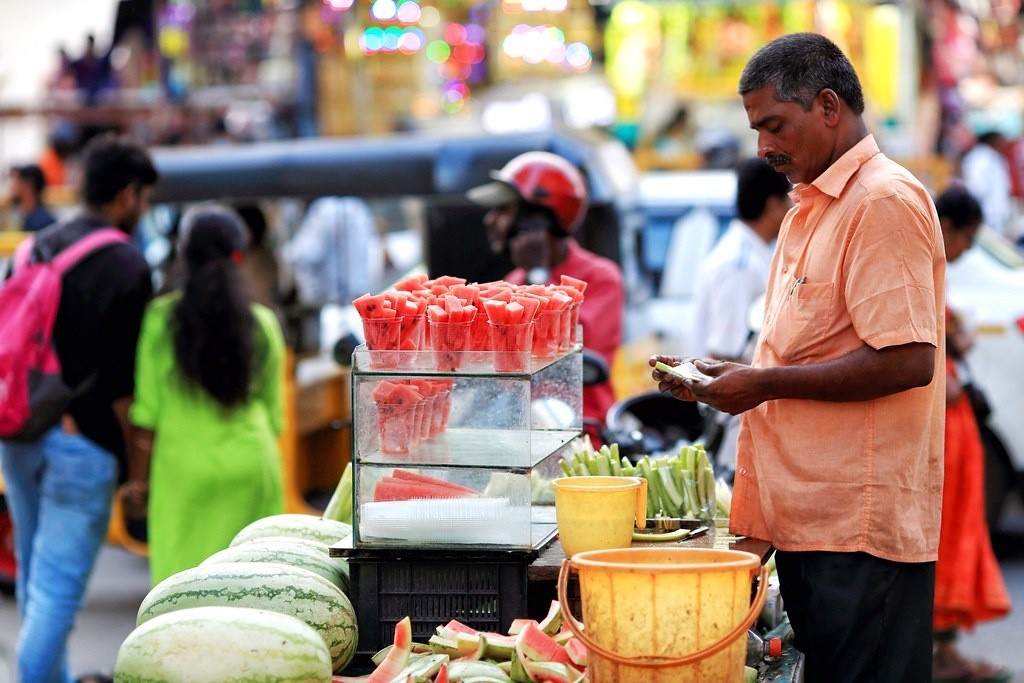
top-left (0, 140), bottom-right (159, 683)
top-left (936, 131), bottom-right (1024, 632)
top-left (464, 151), bottom-right (625, 451)
top-left (648, 31), bottom-right (946, 683)
top-left (0, 165), bottom-right (56, 231)
top-left (663, 157), bottom-right (795, 483)
top-left (127, 202), bottom-right (283, 587)
top-left (280, 191), bottom-right (391, 355)
top-left (70, 35), bottom-right (111, 108)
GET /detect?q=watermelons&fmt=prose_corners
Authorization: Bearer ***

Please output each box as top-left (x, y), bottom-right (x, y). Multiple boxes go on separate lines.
top-left (353, 273), bottom-right (586, 501)
top-left (112, 462), bottom-right (357, 683)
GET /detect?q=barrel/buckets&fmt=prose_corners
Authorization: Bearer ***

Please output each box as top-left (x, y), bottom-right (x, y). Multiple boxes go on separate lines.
top-left (557, 548), bottom-right (770, 683)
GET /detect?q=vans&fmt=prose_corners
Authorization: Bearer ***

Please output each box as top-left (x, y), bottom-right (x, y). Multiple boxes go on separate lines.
top-left (140, 114), bottom-right (741, 348)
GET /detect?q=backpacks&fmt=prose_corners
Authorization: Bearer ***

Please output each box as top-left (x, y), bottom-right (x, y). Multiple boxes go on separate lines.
top-left (0, 229), bottom-right (127, 446)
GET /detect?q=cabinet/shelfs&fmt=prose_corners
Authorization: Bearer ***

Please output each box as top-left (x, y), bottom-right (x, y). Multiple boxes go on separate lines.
top-left (349, 324), bottom-right (585, 551)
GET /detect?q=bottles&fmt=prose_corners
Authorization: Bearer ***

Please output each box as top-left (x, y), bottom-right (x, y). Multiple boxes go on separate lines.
top-left (746, 625), bottom-right (783, 666)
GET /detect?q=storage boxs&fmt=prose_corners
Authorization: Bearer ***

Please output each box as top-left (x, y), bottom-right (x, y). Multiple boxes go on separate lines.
top-left (345, 555), bottom-right (571, 671)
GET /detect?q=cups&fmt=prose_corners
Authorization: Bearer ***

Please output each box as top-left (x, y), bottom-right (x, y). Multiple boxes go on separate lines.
top-left (399, 302), bottom-right (580, 373)
top-left (362, 317), bottom-right (400, 368)
top-left (375, 389), bottom-right (452, 456)
top-left (549, 474), bottom-right (648, 558)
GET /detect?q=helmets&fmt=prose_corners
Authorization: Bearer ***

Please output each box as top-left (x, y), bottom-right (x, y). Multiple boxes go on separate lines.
top-left (466, 152), bottom-right (587, 235)
